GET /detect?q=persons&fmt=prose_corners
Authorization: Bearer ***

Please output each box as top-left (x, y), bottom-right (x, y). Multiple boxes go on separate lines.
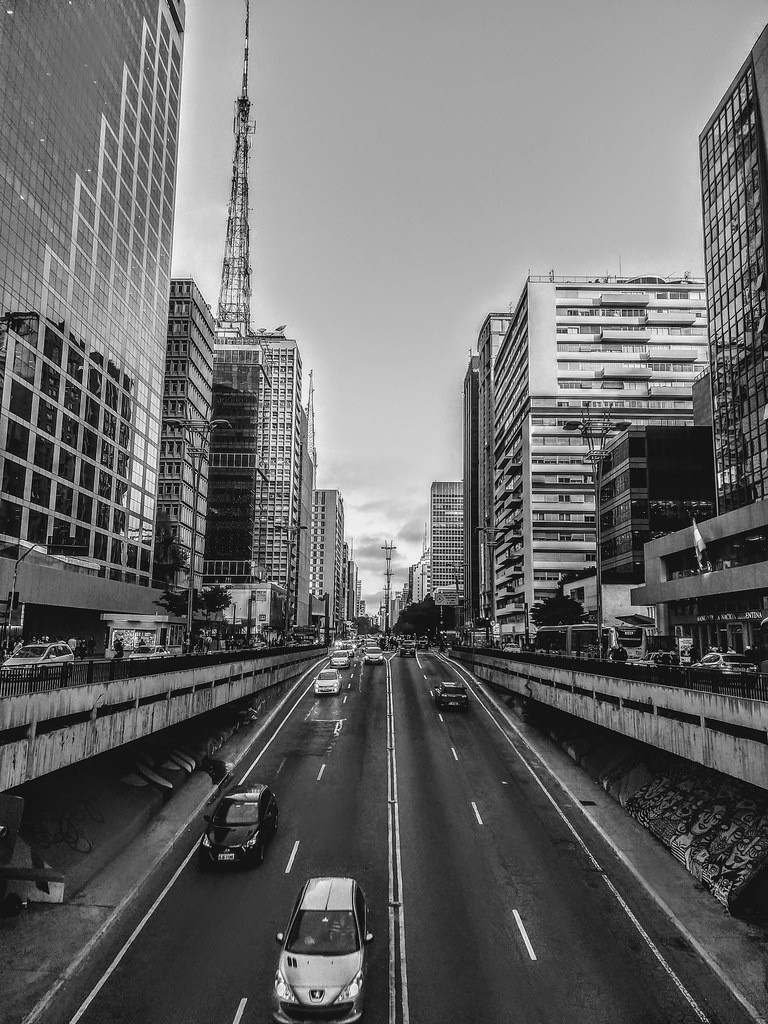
top-left (244, 804), bottom-right (259, 818)
top-left (0, 635), bottom-right (283, 665)
top-left (330, 911), bottom-right (356, 949)
top-left (607, 642), bottom-right (629, 664)
top-left (653, 644), bottom-right (763, 673)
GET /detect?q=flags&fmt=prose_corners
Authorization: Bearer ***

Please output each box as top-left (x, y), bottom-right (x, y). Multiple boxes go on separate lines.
top-left (693, 517), bottom-right (708, 571)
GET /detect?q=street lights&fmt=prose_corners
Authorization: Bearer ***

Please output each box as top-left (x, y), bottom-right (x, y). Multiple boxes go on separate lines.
top-left (474, 526), bottom-right (509, 647)
top-left (458, 595), bottom-right (476, 669)
top-left (563, 401), bottom-right (631, 672)
top-left (380, 540), bottom-right (397, 638)
top-left (447, 562), bottom-right (469, 627)
top-left (163, 417), bottom-right (232, 660)
top-left (273, 522), bottom-right (308, 638)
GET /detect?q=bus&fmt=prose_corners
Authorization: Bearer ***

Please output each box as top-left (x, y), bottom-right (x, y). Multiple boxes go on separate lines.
top-left (533, 623), bottom-right (648, 668)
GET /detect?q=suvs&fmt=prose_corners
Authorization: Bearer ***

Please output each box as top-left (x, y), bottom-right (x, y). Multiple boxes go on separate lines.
top-left (637, 651), bottom-right (680, 667)
top-left (434, 680), bottom-right (470, 712)
top-left (685, 651), bottom-right (759, 688)
top-left (271, 876), bottom-right (371, 1024)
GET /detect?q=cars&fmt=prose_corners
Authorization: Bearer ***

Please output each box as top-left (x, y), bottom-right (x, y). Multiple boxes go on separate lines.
top-left (365, 646), bottom-right (384, 665)
top-left (503, 642), bottom-right (520, 654)
top-left (201, 784), bottom-right (278, 870)
top-left (0, 642), bottom-right (75, 681)
top-left (313, 669), bottom-right (343, 697)
top-left (127, 645), bottom-right (171, 663)
top-left (330, 650), bottom-right (351, 669)
top-left (336, 633), bottom-right (430, 657)
